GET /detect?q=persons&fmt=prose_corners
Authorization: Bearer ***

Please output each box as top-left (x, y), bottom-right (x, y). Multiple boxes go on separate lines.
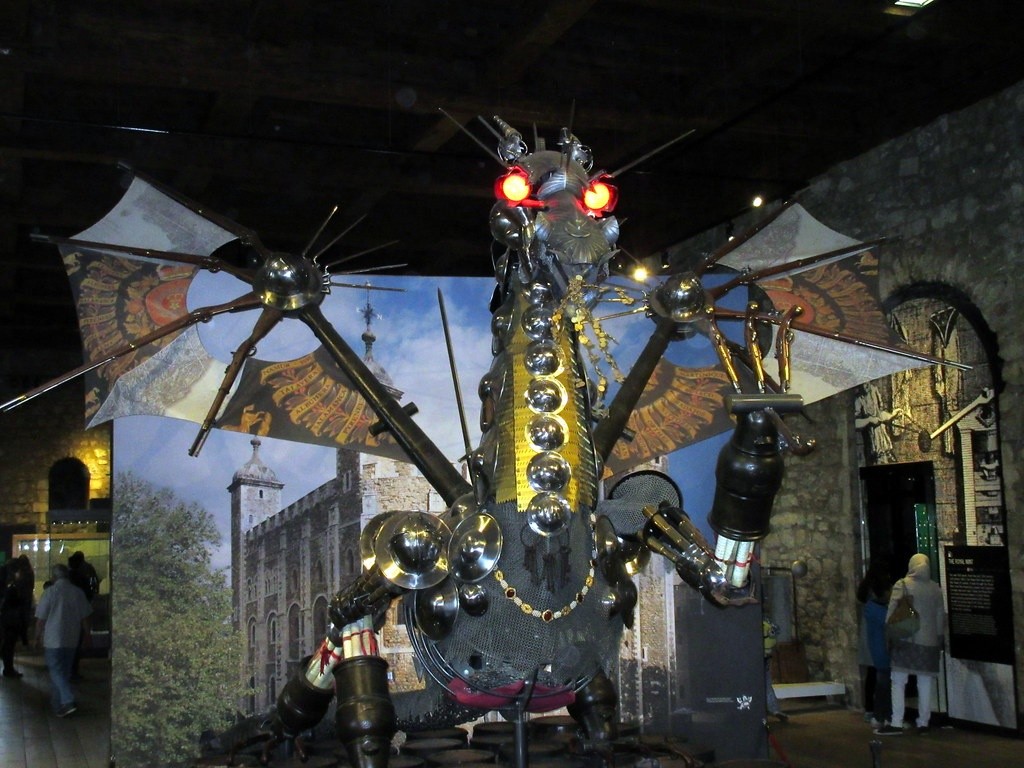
top-left (873, 553), bottom-right (944, 734)
top-left (0, 554), bottom-right (34, 678)
top-left (31, 564), bottom-right (93, 716)
top-left (855, 547), bottom-right (910, 729)
top-left (69, 551), bottom-right (100, 646)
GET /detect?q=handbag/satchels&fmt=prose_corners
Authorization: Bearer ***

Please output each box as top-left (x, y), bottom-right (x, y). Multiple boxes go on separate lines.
top-left (886, 578), bottom-right (920, 640)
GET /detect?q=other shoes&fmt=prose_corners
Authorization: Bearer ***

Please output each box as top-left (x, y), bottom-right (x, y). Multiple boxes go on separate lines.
top-left (57, 702), bottom-right (78, 717)
top-left (865, 711), bottom-right (873, 721)
top-left (4, 670), bottom-right (22, 678)
top-left (918, 725), bottom-right (929, 735)
top-left (873, 724), bottom-right (903, 736)
top-left (870, 717), bottom-right (885, 728)
